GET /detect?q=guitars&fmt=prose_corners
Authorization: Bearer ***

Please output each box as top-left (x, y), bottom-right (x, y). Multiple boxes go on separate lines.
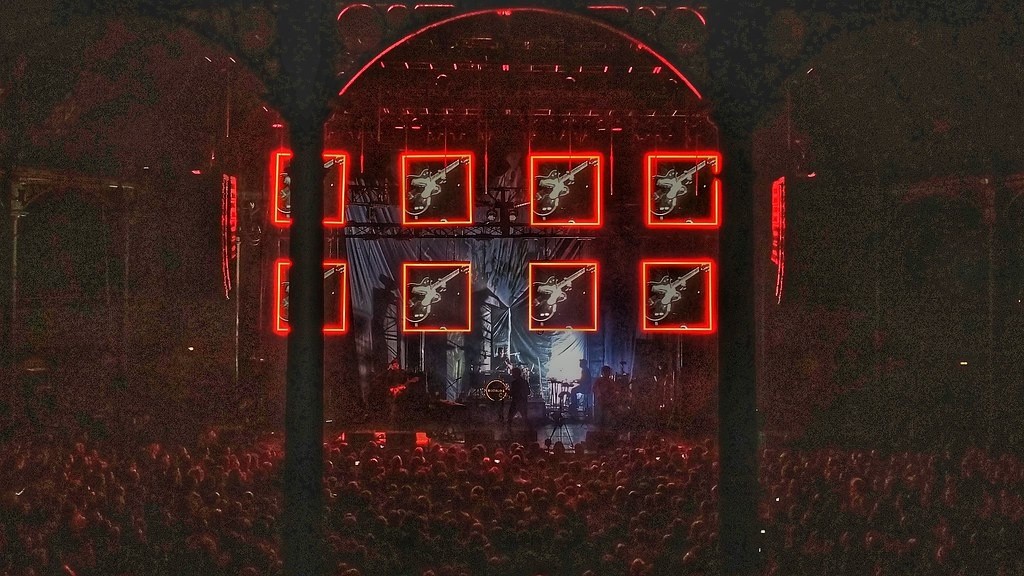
top-left (279, 265), bottom-right (344, 322)
top-left (646, 264), bottom-right (709, 322)
top-left (650, 158), bottom-right (716, 217)
top-left (389, 377), bottom-right (418, 396)
top-left (531, 265), bottom-right (596, 322)
top-left (405, 266), bottom-right (469, 323)
top-left (533, 159), bottom-right (599, 217)
top-left (405, 156), bottom-right (469, 215)
top-left (277, 155), bottom-right (344, 215)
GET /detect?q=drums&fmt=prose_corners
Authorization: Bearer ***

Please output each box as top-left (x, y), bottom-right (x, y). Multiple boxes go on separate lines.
top-left (485, 380), bottom-right (507, 402)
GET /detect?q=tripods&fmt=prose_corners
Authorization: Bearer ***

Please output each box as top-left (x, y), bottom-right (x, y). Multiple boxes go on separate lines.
top-left (549, 396), bottom-right (574, 448)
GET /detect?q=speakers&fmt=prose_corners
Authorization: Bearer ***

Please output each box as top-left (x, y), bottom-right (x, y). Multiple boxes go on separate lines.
top-left (465, 430), bottom-right (494, 445)
top-left (586, 431), bottom-right (621, 450)
top-left (510, 431), bottom-right (537, 442)
top-left (346, 433), bottom-right (378, 449)
top-left (385, 431), bottom-right (416, 447)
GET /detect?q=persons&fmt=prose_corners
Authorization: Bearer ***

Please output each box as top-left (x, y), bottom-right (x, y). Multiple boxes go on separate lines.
top-left (0, 347), bottom-right (1023, 576)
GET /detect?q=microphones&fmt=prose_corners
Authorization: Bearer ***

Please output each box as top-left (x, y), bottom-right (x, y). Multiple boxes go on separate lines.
top-left (455, 377), bottom-right (462, 380)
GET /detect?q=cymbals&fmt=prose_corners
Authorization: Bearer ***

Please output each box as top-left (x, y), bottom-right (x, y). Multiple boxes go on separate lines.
top-left (474, 363), bottom-right (489, 366)
top-left (515, 363), bottom-right (528, 366)
